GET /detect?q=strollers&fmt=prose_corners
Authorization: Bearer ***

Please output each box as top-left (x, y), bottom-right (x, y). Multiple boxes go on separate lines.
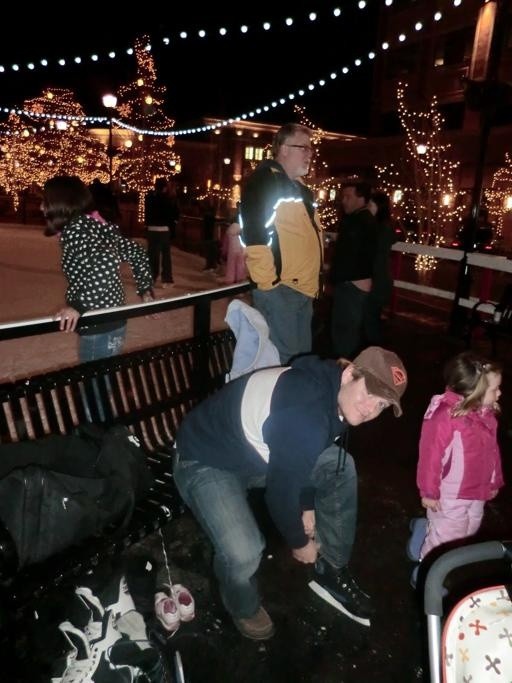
top-left (412, 535), bottom-right (510, 680)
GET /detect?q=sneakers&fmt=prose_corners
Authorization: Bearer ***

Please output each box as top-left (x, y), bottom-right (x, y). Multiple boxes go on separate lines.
top-left (50, 575), bottom-right (152, 683)
top-left (406, 517), bottom-right (428, 562)
top-left (152, 591), bottom-right (181, 631)
top-left (170, 583), bottom-right (196, 623)
top-left (306, 555), bottom-right (375, 628)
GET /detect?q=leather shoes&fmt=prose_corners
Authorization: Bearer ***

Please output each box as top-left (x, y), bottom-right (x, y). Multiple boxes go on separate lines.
top-left (230, 605), bottom-right (276, 643)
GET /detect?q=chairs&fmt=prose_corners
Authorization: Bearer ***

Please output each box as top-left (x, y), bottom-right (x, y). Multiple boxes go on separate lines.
top-left (424, 541), bottom-right (511, 682)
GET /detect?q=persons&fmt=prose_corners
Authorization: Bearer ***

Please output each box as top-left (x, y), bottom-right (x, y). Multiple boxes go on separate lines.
top-left (171, 347), bottom-right (408, 642)
top-left (328, 177), bottom-right (374, 352)
top-left (222, 220), bottom-right (246, 298)
top-left (242, 123), bottom-right (322, 361)
top-left (407, 346), bottom-right (501, 600)
top-left (200, 199), bottom-right (218, 273)
top-left (144, 177), bottom-right (174, 289)
top-left (367, 192), bottom-right (396, 345)
top-left (39, 175), bottom-right (157, 421)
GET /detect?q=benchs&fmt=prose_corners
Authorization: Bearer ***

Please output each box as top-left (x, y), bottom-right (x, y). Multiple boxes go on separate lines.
top-left (2, 328), bottom-right (238, 610)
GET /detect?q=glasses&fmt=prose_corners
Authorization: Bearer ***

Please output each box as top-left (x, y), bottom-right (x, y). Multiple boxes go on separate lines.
top-left (285, 143), bottom-right (315, 152)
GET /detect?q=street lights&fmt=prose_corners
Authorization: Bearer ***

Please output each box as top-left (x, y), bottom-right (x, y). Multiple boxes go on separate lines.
top-left (100, 91), bottom-right (118, 219)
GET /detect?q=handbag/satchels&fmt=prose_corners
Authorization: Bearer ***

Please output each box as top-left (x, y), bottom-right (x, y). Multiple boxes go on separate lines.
top-left (1, 461), bottom-right (113, 564)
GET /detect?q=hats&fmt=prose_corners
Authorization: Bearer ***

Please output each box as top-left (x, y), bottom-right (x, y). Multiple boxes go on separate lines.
top-left (350, 345), bottom-right (409, 419)
